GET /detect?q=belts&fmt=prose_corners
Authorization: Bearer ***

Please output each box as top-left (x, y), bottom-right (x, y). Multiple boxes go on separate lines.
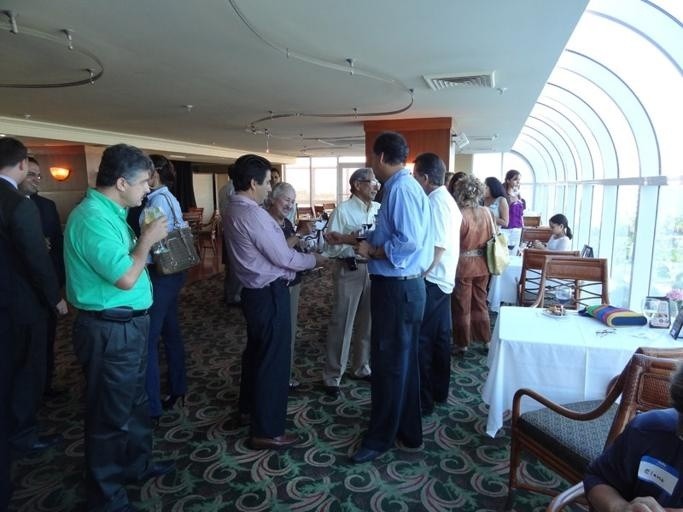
top-left (336, 256), bottom-right (366, 264)
top-left (77, 308), bottom-right (151, 320)
top-left (368, 274), bottom-right (421, 281)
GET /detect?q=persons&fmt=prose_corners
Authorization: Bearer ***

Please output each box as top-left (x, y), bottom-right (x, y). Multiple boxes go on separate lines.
top-left (582, 368), bottom-right (683, 512)
top-left (533, 214), bottom-right (572, 252)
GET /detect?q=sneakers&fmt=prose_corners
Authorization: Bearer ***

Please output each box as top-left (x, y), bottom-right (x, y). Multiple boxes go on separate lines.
top-left (290, 379), bottom-right (313, 392)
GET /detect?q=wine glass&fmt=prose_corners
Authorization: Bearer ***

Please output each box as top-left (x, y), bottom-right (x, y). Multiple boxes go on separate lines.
top-left (145, 206), bottom-right (172, 255)
top-left (555, 286), bottom-right (572, 321)
top-left (507, 237), bottom-right (530, 262)
top-left (297, 239), bottom-right (326, 255)
top-left (353, 215), bottom-right (374, 260)
top-left (640, 298), bottom-right (659, 333)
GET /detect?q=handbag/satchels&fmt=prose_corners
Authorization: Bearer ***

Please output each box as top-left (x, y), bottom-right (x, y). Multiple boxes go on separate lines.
top-left (582, 302), bottom-right (647, 326)
top-left (485, 232), bottom-right (510, 276)
top-left (149, 227), bottom-right (201, 278)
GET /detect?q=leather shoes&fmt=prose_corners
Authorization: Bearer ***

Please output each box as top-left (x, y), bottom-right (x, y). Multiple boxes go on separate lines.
top-left (349, 446), bottom-right (382, 463)
top-left (350, 373), bottom-right (372, 384)
top-left (325, 386), bottom-right (340, 396)
top-left (24, 433), bottom-right (64, 450)
top-left (148, 458), bottom-right (176, 477)
top-left (237, 410), bottom-right (252, 426)
top-left (246, 431), bottom-right (299, 450)
top-left (227, 298), bottom-right (242, 308)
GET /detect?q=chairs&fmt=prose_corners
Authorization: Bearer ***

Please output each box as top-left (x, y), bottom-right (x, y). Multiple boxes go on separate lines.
top-left (507, 347), bottom-right (682, 512)
top-left (299, 204), bottom-right (335, 251)
top-left (520, 216), bottom-right (609, 311)
top-left (184, 208), bottom-right (221, 282)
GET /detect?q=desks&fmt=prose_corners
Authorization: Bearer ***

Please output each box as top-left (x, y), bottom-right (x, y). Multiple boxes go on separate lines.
top-left (487, 256), bottom-right (576, 310)
top-left (482, 304), bottom-right (683, 438)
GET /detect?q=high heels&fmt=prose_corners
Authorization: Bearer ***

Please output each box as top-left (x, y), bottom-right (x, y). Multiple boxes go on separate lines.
top-left (451, 346), bottom-right (466, 356)
top-left (151, 414), bottom-right (161, 426)
top-left (160, 393), bottom-right (185, 409)
top-left (483, 342), bottom-right (491, 352)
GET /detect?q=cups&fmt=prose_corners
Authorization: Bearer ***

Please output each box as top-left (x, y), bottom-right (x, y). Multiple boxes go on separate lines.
top-left (298, 214), bottom-right (310, 225)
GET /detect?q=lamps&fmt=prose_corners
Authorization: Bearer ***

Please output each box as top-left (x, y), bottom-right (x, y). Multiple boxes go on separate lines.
top-left (45, 162), bottom-right (70, 183)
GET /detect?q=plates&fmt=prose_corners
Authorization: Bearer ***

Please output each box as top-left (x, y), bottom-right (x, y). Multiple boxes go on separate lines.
top-left (543, 309), bottom-right (570, 318)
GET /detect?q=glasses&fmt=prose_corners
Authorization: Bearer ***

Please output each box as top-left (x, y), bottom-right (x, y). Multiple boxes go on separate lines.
top-left (358, 179), bottom-right (381, 187)
